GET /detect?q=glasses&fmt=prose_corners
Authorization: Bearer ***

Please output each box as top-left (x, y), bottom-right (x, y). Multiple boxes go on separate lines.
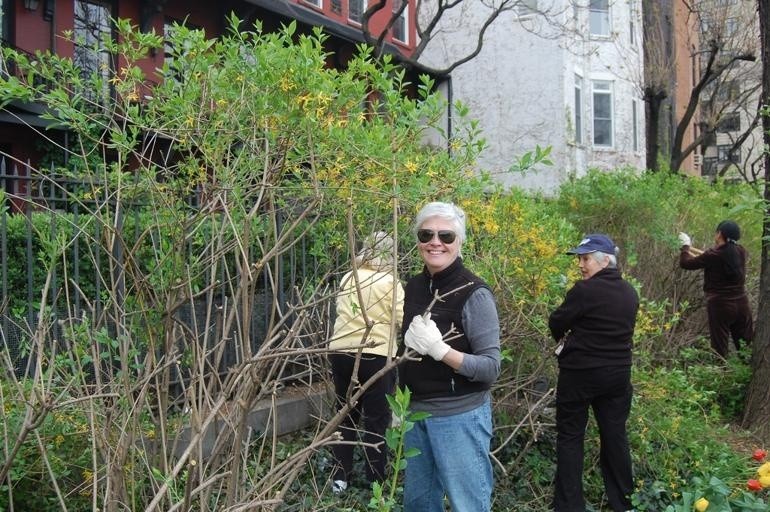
top-left (417, 229), bottom-right (456, 244)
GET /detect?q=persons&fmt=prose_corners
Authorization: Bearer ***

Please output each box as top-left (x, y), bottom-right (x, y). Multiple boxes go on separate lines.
top-left (549, 234), bottom-right (640, 511)
top-left (328, 230), bottom-right (405, 496)
top-left (678, 220), bottom-right (758, 370)
top-left (393, 200), bottom-right (502, 511)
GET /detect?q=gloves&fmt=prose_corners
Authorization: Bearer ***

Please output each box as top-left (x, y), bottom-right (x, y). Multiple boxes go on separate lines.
top-left (403, 312), bottom-right (451, 361)
top-left (678, 231), bottom-right (690, 249)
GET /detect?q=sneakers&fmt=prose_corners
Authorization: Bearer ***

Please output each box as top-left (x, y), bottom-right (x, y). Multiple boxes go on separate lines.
top-left (332, 475), bottom-right (346, 497)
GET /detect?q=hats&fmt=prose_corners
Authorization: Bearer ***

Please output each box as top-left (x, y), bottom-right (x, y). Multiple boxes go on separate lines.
top-left (566, 234), bottom-right (619, 255)
